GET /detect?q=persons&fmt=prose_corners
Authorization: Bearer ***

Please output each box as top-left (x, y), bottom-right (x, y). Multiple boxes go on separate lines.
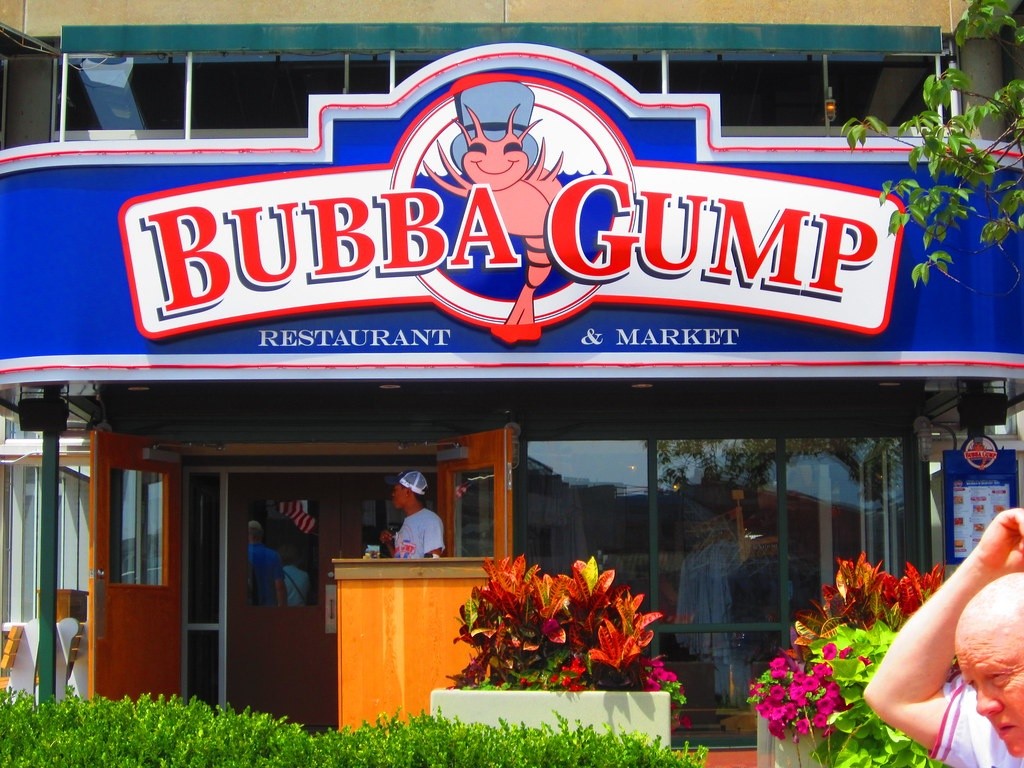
top-left (278, 543), bottom-right (310, 607)
top-left (864, 508), bottom-right (1024, 768)
top-left (378, 470), bottom-right (445, 558)
top-left (248, 521), bottom-right (287, 607)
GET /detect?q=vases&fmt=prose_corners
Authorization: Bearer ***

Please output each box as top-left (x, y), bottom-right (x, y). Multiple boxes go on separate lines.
top-left (757, 710), bottom-right (831, 768)
top-left (431, 687), bottom-right (671, 752)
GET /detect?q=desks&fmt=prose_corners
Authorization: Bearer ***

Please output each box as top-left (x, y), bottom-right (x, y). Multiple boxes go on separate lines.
top-left (333, 558), bottom-right (494, 735)
top-left (36, 589), bottom-right (90, 623)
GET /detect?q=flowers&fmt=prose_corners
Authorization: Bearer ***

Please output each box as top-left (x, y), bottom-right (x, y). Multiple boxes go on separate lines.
top-left (446, 553), bottom-right (692, 735)
top-left (746, 551), bottom-right (956, 768)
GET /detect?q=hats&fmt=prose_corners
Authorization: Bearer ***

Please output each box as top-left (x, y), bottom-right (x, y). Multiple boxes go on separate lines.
top-left (383, 469), bottom-right (428, 495)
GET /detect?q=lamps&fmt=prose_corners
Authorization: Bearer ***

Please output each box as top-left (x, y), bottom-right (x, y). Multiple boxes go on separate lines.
top-left (825, 86), bottom-right (837, 122)
top-left (68, 396), bottom-right (101, 430)
top-left (17, 381), bottom-right (69, 432)
top-left (956, 376), bottom-right (1008, 425)
top-left (914, 416), bottom-right (932, 462)
top-left (505, 422), bottom-right (520, 469)
